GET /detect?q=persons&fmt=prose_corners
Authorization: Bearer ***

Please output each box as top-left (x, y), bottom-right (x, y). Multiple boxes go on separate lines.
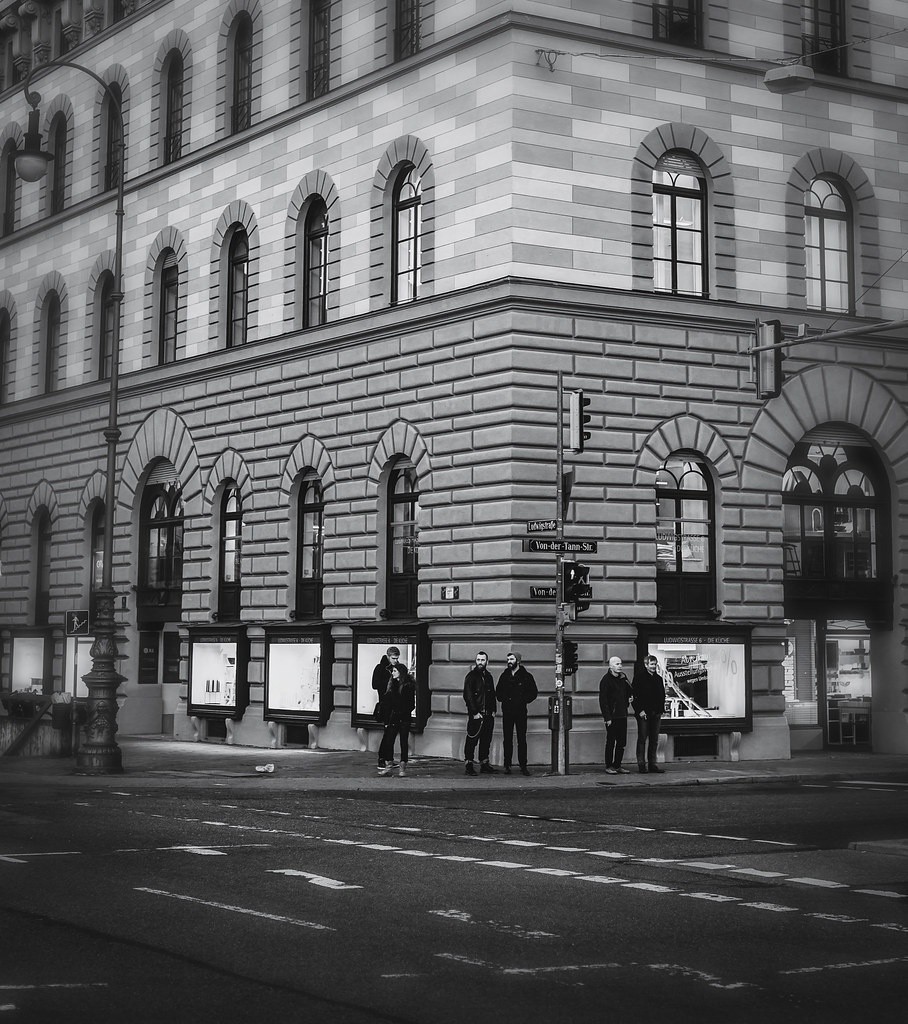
top-left (496, 652), bottom-right (538, 776)
top-left (463, 651), bottom-right (499, 776)
top-left (372, 647), bottom-right (400, 702)
top-left (631, 655), bottom-right (666, 774)
top-left (599, 656), bottom-right (633, 773)
top-left (378, 664), bottom-right (416, 776)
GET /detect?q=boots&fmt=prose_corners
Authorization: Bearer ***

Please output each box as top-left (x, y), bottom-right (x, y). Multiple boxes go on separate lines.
top-left (378, 760), bottom-right (394, 777)
top-left (399, 761), bottom-right (407, 777)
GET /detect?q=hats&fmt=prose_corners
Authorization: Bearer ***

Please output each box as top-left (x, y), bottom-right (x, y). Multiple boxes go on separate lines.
top-left (509, 652), bottom-right (521, 664)
top-left (395, 664), bottom-right (408, 674)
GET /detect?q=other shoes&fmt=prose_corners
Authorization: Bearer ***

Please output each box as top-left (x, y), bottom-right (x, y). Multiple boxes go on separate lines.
top-left (615, 767), bottom-right (630, 774)
top-left (648, 764), bottom-right (664, 773)
top-left (522, 769), bottom-right (532, 776)
top-left (639, 766), bottom-right (648, 773)
top-left (605, 766), bottom-right (618, 774)
top-left (505, 767), bottom-right (512, 774)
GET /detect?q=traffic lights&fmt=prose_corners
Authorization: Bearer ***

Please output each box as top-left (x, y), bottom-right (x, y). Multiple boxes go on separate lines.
top-left (569, 388), bottom-right (592, 454)
top-left (563, 563), bottom-right (581, 603)
top-left (758, 320), bottom-right (788, 402)
top-left (562, 639), bottom-right (578, 676)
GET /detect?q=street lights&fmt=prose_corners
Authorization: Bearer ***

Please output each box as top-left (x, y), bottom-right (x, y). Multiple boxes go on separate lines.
top-left (13, 61), bottom-right (125, 777)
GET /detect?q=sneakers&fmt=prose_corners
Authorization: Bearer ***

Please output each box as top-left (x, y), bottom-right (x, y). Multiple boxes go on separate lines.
top-left (377, 758), bottom-right (386, 770)
top-left (480, 763), bottom-right (499, 774)
top-left (392, 764), bottom-right (399, 767)
top-left (465, 762), bottom-right (477, 776)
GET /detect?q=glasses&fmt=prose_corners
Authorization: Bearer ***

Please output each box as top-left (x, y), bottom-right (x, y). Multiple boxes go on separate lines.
top-left (390, 656), bottom-right (400, 659)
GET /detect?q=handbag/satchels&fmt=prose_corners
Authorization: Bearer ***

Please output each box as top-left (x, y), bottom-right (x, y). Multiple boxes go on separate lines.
top-left (373, 703), bottom-right (385, 722)
